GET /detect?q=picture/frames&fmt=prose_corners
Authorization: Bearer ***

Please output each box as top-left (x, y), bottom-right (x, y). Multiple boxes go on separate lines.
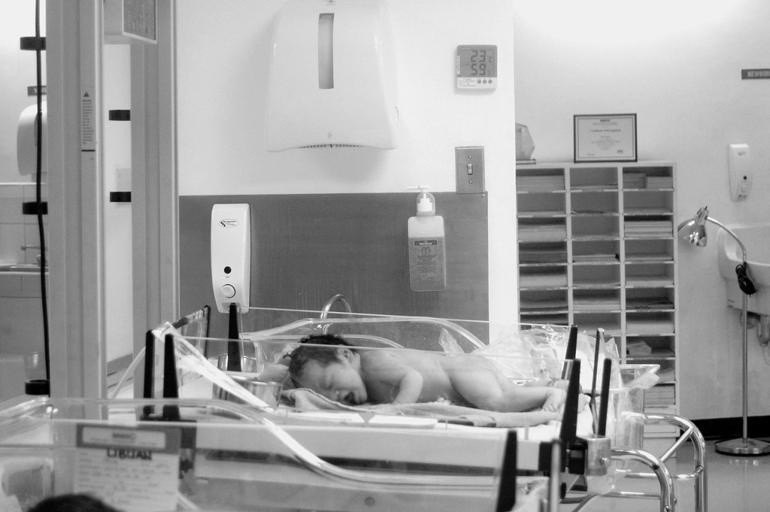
top-left (571, 112), bottom-right (638, 164)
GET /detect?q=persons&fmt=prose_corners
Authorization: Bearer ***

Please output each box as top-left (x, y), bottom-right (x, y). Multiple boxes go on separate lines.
top-left (284, 331), bottom-right (585, 414)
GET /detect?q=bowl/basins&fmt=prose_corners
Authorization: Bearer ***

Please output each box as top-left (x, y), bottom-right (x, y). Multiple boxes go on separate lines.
top-left (212, 373), bottom-right (282, 409)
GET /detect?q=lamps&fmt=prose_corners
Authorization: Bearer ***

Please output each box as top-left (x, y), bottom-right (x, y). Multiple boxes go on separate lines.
top-left (679, 203), bottom-right (769, 458)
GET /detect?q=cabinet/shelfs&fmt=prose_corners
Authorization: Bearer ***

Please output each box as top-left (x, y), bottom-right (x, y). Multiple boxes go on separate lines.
top-left (517, 160), bottom-right (678, 480)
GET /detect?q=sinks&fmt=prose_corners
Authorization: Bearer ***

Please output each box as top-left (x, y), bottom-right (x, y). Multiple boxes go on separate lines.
top-left (716, 225), bottom-right (769, 317)
